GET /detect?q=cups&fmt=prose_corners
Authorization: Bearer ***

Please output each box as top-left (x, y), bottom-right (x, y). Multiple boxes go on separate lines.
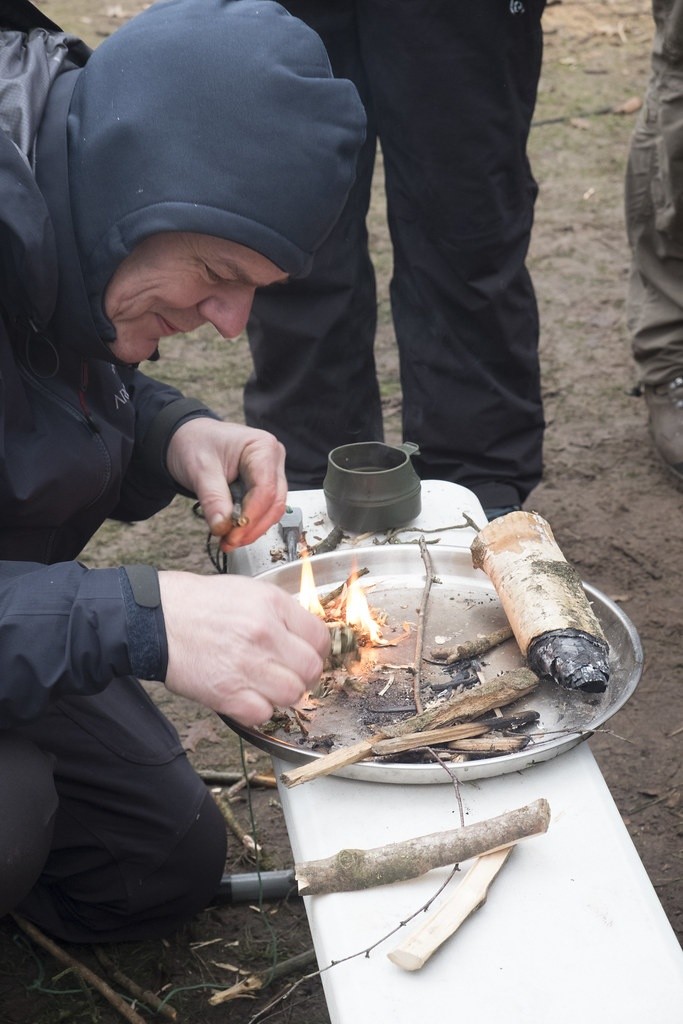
top-left (323, 441), bottom-right (422, 531)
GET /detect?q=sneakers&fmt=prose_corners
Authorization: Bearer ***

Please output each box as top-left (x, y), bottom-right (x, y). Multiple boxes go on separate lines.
top-left (645, 378), bottom-right (683, 484)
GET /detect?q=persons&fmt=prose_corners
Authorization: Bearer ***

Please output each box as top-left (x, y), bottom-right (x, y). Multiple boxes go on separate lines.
top-left (2, 1), bottom-right (371, 943)
top-left (242, 0), bottom-right (550, 522)
top-left (624, 0), bottom-right (682, 485)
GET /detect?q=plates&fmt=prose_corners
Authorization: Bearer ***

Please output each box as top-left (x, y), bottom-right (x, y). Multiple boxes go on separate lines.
top-left (215, 539), bottom-right (644, 785)
